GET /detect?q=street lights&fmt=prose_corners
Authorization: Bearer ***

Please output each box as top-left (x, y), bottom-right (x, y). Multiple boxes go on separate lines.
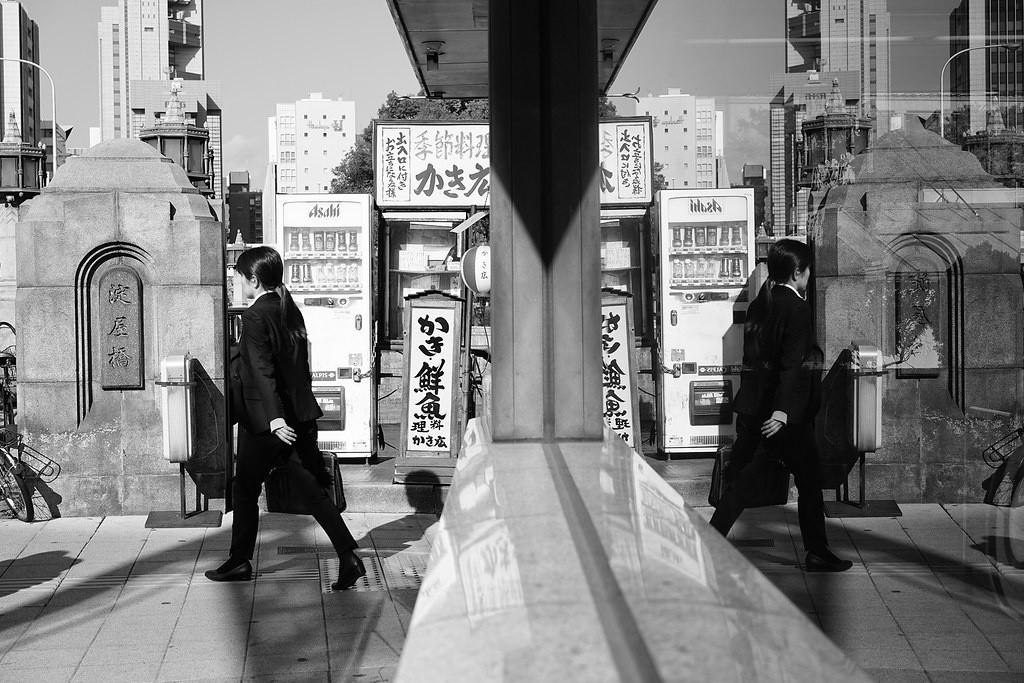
top-left (940, 42), bottom-right (1020, 138)
top-left (39, 142), bottom-right (46, 186)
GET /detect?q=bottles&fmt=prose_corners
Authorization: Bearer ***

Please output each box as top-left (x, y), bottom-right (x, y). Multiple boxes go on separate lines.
top-left (442, 244), bottom-right (457, 265)
top-left (676, 263), bottom-right (714, 278)
top-left (317, 267), bottom-right (356, 283)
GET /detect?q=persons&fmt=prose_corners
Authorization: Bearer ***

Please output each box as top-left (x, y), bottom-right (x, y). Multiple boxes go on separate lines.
top-left (710, 238), bottom-right (853, 572)
top-left (204, 245), bottom-right (365, 592)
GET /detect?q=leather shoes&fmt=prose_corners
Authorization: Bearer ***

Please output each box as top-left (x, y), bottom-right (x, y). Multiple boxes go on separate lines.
top-left (805, 549), bottom-right (853, 572)
top-left (331, 553), bottom-right (366, 590)
top-left (204, 561), bottom-right (252, 581)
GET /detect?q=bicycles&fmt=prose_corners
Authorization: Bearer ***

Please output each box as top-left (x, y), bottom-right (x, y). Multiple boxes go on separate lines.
top-left (0, 430), bottom-right (35, 523)
top-left (0, 344), bottom-right (16, 428)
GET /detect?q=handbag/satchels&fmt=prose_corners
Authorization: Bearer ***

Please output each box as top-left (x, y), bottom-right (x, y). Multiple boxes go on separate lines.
top-left (707, 444), bottom-right (791, 509)
top-left (265, 451), bottom-right (347, 515)
